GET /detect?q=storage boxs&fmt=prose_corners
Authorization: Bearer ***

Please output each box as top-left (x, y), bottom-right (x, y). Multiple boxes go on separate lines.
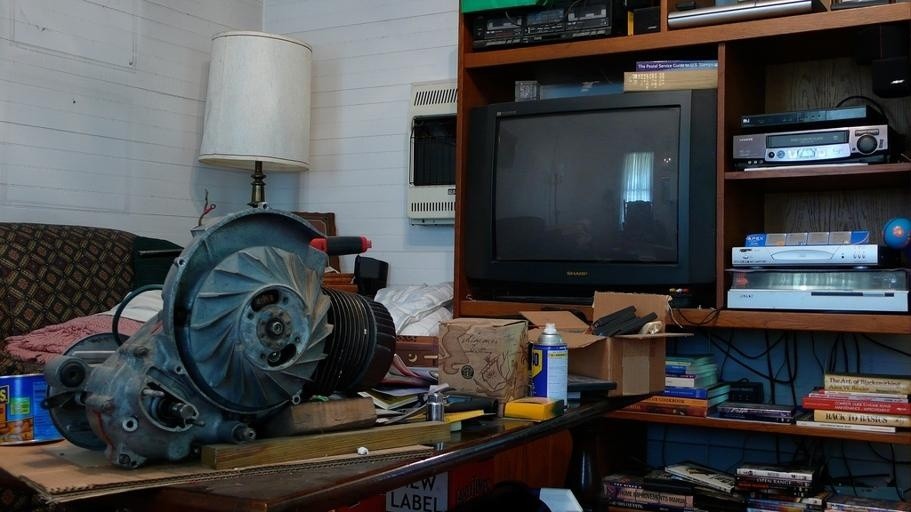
top-left (435, 317), bottom-right (530, 405)
top-left (515, 288), bottom-right (691, 396)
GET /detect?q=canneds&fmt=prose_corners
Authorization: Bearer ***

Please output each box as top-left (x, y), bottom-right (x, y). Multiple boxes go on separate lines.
top-left (0, 372), bottom-right (64, 446)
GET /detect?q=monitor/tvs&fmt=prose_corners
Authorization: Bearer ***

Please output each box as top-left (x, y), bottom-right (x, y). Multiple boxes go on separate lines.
top-left (464, 89), bottom-right (716, 306)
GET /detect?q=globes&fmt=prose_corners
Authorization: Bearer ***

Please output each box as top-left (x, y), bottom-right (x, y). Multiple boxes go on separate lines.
top-left (882, 216), bottom-right (911, 269)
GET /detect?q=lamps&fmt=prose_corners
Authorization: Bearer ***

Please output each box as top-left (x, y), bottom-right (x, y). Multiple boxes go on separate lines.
top-left (194, 28), bottom-right (317, 212)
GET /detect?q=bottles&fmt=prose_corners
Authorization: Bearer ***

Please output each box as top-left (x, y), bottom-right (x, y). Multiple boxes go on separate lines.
top-left (531, 322), bottom-right (570, 412)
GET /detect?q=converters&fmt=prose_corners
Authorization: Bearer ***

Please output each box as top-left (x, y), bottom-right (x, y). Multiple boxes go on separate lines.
top-left (730, 383), bottom-right (763, 401)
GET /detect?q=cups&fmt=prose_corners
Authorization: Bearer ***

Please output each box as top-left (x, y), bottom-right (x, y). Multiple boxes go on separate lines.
top-left (427, 394), bottom-right (446, 426)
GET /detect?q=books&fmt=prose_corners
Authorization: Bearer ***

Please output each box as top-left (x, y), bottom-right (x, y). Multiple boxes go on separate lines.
top-left (357, 352), bottom-right (439, 424)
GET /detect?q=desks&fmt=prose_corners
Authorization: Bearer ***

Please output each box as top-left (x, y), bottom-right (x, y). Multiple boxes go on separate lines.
top-left (146, 388), bottom-right (657, 510)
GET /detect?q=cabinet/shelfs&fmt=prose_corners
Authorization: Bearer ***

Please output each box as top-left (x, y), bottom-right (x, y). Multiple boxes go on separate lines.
top-left (447, 0), bottom-right (911, 512)
